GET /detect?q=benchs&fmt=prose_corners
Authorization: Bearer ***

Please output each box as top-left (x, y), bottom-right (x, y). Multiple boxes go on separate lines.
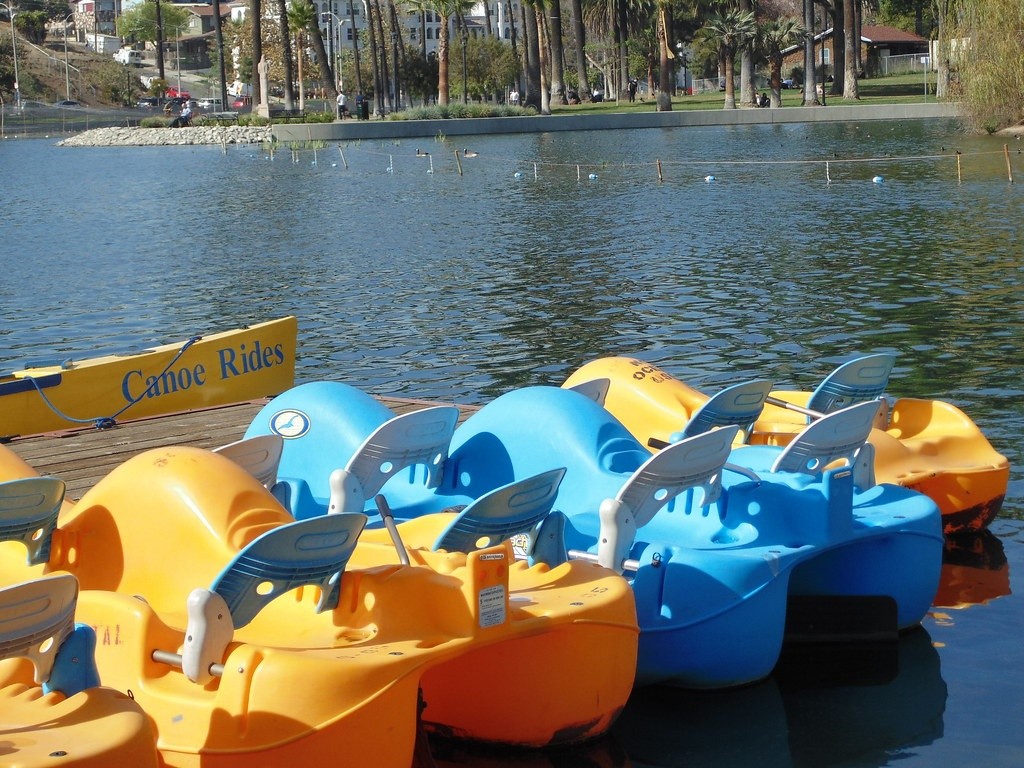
top-left (375, 105), bottom-right (400, 118)
top-left (268, 109), bottom-right (308, 125)
top-left (205, 111), bottom-right (239, 127)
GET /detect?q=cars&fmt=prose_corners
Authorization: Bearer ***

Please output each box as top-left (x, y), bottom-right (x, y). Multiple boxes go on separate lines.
top-left (196, 98), bottom-right (220, 109)
top-left (165, 85), bottom-right (190, 102)
top-left (232, 97), bottom-right (251, 109)
top-left (136, 97), bottom-right (168, 110)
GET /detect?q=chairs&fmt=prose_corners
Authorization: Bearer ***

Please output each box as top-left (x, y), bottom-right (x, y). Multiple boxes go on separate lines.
top-left (0, 350), bottom-right (900, 704)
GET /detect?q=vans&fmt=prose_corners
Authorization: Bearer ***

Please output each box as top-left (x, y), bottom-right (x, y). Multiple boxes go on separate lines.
top-left (116, 50), bottom-right (141, 67)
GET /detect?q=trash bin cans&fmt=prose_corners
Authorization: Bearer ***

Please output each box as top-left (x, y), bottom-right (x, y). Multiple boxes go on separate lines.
top-left (356, 95), bottom-right (369, 120)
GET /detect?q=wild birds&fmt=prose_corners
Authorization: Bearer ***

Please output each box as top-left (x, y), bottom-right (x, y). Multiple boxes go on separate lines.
top-left (463, 148), bottom-right (479, 159)
top-left (415, 148), bottom-right (431, 157)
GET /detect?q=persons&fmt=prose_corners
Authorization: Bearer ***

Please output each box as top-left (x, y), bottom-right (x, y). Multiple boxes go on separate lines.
top-left (337, 91), bottom-right (346, 120)
top-left (167, 103), bottom-right (191, 128)
top-left (163, 100), bottom-right (172, 117)
top-left (186, 98), bottom-right (191, 108)
top-left (594, 88), bottom-right (599, 96)
top-left (828, 75), bottom-right (833, 82)
top-left (720, 77), bottom-right (726, 91)
top-left (510, 89), bottom-right (519, 105)
top-left (755, 93), bottom-right (770, 107)
top-left (355, 91), bottom-right (366, 106)
top-left (628, 78), bottom-right (639, 103)
top-left (781, 78), bottom-right (798, 89)
